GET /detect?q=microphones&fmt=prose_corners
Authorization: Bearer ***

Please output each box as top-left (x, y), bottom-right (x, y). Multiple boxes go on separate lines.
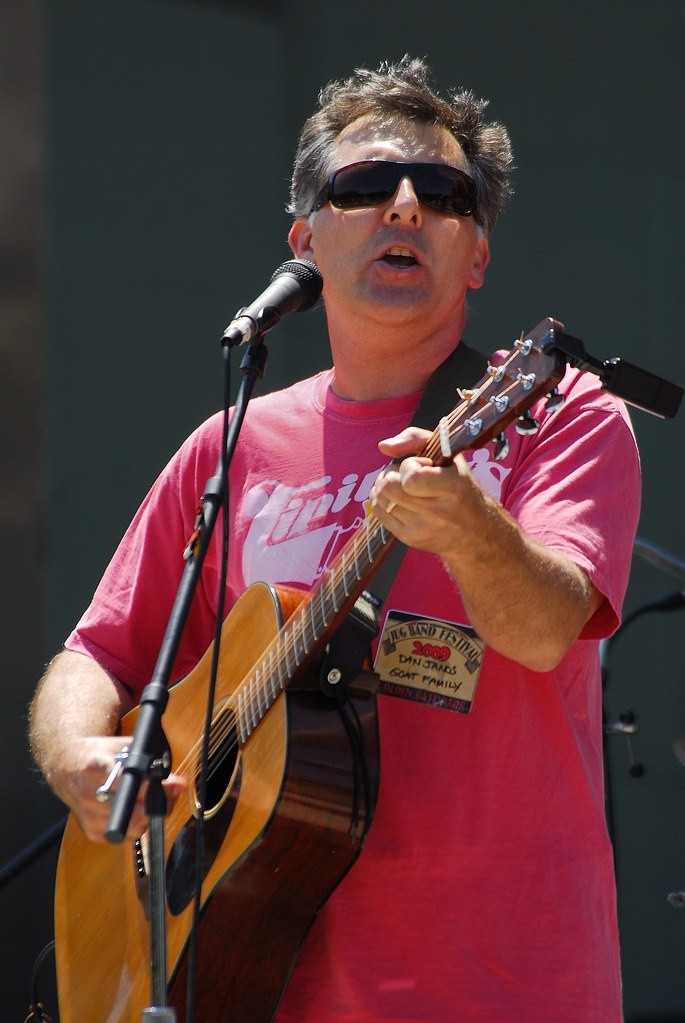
top-left (218, 259), bottom-right (324, 350)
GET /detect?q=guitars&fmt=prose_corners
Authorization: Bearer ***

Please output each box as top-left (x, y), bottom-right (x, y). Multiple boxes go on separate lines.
top-left (53, 316), bottom-right (566, 1023)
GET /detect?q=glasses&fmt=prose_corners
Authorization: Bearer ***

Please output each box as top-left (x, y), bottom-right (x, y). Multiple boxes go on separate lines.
top-left (308, 161), bottom-right (483, 226)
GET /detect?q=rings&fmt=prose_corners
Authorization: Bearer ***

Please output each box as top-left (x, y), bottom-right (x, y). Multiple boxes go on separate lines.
top-left (386, 501), bottom-right (396, 513)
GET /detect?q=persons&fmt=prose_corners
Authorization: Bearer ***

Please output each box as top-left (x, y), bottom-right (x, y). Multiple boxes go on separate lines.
top-left (27, 52), bottom-right (643, 1022)
